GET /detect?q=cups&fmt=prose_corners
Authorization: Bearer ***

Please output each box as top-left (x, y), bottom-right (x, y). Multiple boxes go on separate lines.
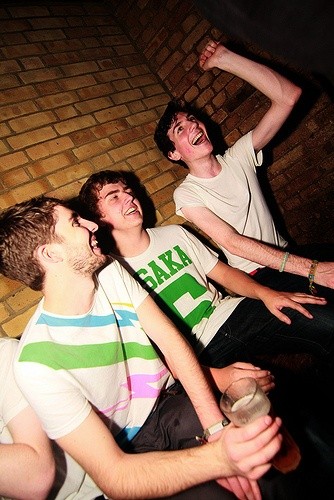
top-left (220, 377), bottom-right (301, 474)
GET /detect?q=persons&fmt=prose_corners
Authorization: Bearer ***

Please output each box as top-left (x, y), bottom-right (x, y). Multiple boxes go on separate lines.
top-left (0, 337), bottom-right (107, 500)
top-left (0, 195), bottom-right (333, 500)
top-left (153, 37), bottom-right (333, 302)
top-left (78, 169), bottom-right (333, 425)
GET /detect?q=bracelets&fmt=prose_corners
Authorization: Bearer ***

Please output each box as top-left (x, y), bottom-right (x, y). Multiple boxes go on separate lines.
top-left (195, 418), bottom-right (230, 443)
top-left (309, 259), bottom-right (318, 294)
top-left (278, 250), bottom-right (290, 272)
